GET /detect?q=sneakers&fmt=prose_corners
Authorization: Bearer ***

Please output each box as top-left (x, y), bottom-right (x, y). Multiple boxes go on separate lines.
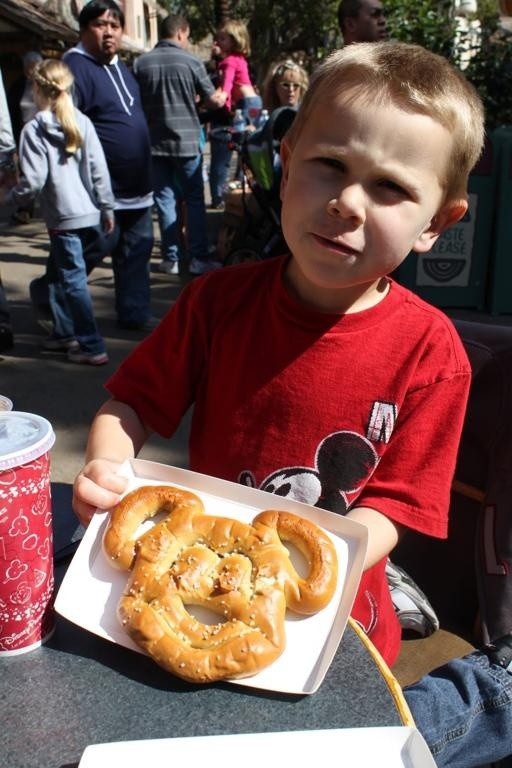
top-left (385, 556), bottom-right (440, 638)
top-left (30, 256), bottom-right (222, 364)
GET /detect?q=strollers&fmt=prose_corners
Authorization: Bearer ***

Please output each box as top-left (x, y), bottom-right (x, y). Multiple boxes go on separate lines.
top-left (209, 104), bottom-right (299, 269)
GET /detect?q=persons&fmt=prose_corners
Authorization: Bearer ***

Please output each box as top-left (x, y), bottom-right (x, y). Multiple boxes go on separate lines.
top-left (1, 1), bottom-right (310, 365)
top-left (336, 0), bottom-right (390, 43)
top-left (71, 40), bottom-right (511, 768)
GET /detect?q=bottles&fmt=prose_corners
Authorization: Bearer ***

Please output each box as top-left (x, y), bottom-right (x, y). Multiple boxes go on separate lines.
top-left (258, 108), bottom-right (270, 130)
top-left (233, 108), bottom-right (246, 134)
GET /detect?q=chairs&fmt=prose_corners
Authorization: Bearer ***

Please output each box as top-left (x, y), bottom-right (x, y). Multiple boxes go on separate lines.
top-left (389, 317), bottom-right (512, 689)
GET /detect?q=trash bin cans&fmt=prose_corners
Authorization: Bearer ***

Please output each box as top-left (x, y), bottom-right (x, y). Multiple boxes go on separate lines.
top-left (397, 168), bottom-right (500, 313)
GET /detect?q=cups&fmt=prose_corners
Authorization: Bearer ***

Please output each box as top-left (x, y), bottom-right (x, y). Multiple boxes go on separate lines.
top-left (0, 409), bottom-right (59, 659)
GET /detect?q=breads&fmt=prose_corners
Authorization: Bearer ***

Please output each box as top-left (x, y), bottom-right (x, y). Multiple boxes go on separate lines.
top-left (103, 485), bottom-right (337, 684)
